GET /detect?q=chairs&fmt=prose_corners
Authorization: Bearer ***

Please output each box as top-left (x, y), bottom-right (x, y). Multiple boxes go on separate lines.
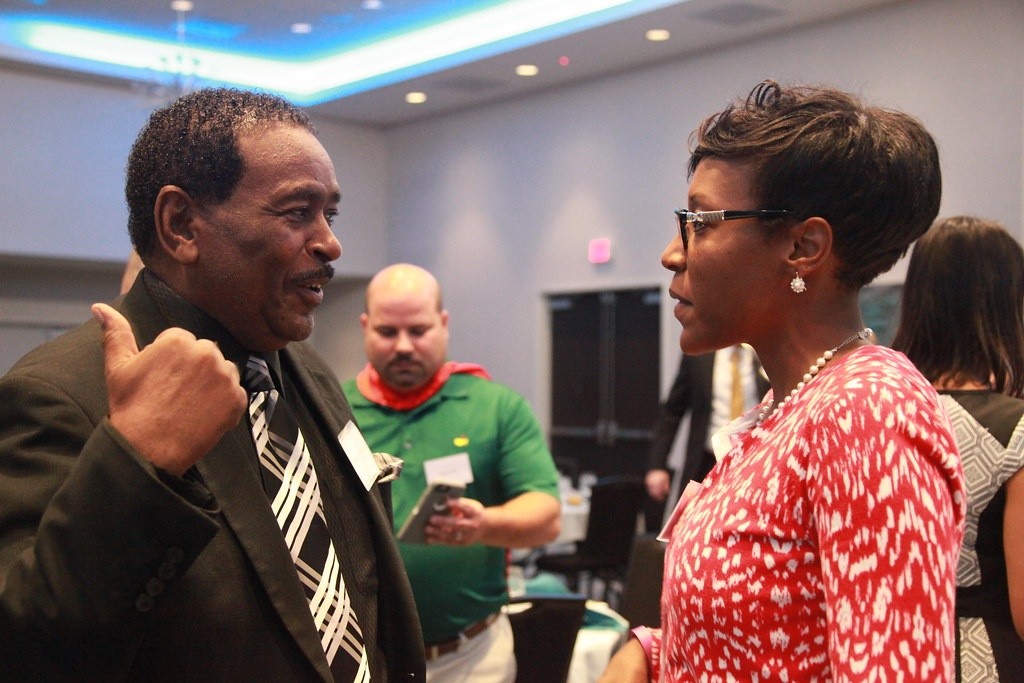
top-left (501, 456), bottom-right (668, 683)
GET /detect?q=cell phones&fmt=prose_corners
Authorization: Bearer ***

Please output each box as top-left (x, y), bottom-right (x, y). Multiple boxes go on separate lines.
top-left (398, 482), bottom-right (466, 543)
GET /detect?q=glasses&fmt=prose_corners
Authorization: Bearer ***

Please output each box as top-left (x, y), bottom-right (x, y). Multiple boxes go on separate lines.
top-left (674, 206), bottom-right (798, 249)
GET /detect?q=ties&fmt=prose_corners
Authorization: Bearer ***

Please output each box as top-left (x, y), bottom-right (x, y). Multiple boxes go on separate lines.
top-left (724, 343), bottom-right (748, 422)
top-left (243, 353), bottom-right (377, 683)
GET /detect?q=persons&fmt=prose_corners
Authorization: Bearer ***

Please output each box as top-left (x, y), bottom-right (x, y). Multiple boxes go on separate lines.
top-left (341, 264), bottom-right (562, 683)
top-left (645, 342), bottom-right (771, 510)
top-left (0, 87), bottom-right (427, 683)
top-left (889, 215), bottom-right (1024, 683)
top-left (604, 80), bottom-right (968, 683)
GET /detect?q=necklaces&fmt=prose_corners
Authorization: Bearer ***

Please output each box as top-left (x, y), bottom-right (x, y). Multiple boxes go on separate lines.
top-left (756, 330), bottom-right (874, 430)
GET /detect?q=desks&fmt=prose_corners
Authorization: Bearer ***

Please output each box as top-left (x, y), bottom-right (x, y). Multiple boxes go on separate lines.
top-left (501, 500), bottom-right (629, 683)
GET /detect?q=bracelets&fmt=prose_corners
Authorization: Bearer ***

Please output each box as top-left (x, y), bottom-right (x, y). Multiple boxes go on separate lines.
top-left (629, 625), bottom-right (653, 662)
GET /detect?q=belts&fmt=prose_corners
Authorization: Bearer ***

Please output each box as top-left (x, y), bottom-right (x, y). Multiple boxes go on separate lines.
top-left (423, 604), bottom-right (503, 660)
